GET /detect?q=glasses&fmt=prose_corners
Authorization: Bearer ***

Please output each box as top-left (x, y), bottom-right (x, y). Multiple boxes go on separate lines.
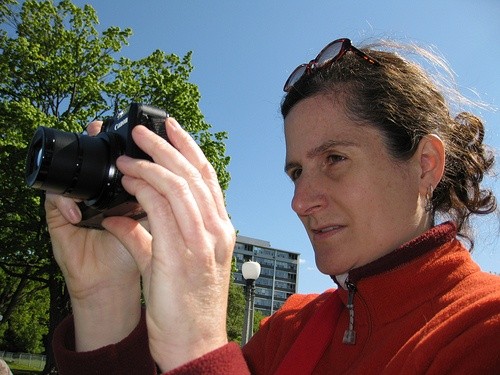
top-left (282, 38), bottom-right (387, 93)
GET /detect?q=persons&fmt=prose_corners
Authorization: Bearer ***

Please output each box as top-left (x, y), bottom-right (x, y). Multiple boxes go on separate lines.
top-left (42, 34), bottom-right (500, 375)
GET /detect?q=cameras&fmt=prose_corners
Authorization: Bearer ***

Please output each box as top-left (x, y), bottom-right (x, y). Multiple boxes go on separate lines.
top-left (24, 103), bottom-right (176, 231)
